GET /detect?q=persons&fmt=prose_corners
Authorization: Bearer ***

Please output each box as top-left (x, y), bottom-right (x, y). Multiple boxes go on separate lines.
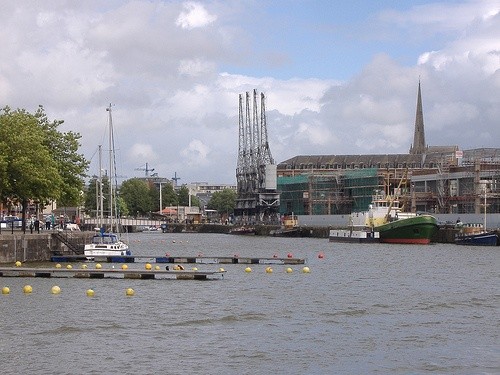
top-left (31, 213), bottom-right (51, 231)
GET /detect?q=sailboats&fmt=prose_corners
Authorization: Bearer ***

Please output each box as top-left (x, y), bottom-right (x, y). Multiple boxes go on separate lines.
top-left (50, 102), bottom-right (136, 262)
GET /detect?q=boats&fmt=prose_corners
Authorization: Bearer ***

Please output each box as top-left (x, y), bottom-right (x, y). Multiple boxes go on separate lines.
top-left (328, 161), bottom-right (441, 244)
top-left (431, 183), bottom-right (500, 246)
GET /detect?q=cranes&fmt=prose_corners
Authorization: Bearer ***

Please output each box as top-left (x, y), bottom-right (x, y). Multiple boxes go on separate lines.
top-left (134, 162), bottom-right (155, 177)
top-left (231, 87), bottom-right (282, 226)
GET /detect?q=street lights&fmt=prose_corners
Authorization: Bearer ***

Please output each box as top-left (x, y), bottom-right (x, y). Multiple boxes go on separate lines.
top-left (153, 182), bottom-right (168, 215)
